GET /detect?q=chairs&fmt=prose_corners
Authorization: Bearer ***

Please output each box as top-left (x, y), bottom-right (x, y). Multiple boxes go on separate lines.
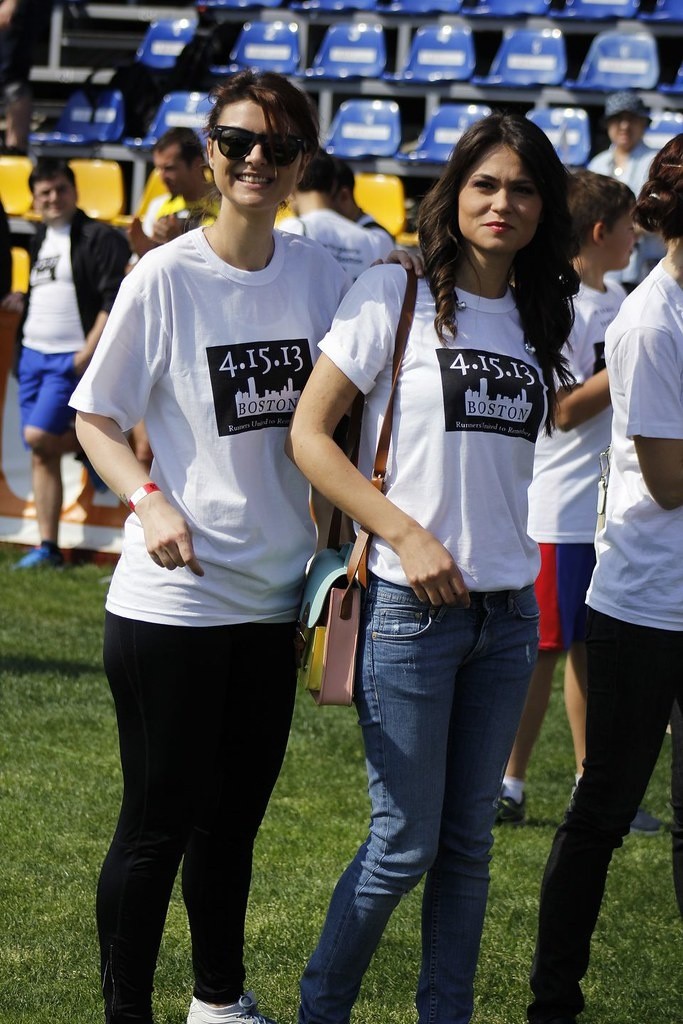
top-left (1, 0), bottom-right (683, 295)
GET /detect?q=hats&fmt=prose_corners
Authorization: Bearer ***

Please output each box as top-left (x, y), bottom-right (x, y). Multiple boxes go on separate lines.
top-left (604, 92), bottom-right (652, 124)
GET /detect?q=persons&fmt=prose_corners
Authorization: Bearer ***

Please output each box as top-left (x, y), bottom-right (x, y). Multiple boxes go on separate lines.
top-left (1, 0), bottom-right (36, 156)
top-left (526, 133), bottom-right (683, 1024)
top-left (125, 129), bottom-right (223, 251)
top-left (579, 90), bottom-right (668, 297)
top-left (12, 157), bottom-right (129, 567)
top-left (63, 76), bottom-right (431, 1024)
top-left (492, 171), bottom-right (667, 836)
top-left (279, 115), bottom-right (579, 1024)
top-left (277, 144), bottom-right (401, 288)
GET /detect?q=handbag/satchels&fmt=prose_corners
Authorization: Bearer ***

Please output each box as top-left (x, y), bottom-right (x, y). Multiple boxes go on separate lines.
top-left (297, 540), bottom-right (362, 705)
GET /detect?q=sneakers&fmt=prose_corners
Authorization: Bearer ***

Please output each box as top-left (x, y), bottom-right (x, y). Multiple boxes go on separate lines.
top-left (74, 449), bottom-right (109, 493)
top-left (186, 990), bottom-right (278, 1024)
top-left (493, 784), bottom-right (530, 824)
top-left (14, 546), bottom-right (63, 570)
top-left (564, 784), bottom-right (666, 836)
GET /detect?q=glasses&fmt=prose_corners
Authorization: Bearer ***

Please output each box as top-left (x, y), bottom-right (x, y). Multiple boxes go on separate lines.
top-left (209, 124), bottom-right (307, 167)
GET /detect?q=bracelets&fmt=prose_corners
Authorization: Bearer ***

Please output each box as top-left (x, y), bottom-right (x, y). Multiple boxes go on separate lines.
top-left (127, 482), bottom-right (161, 511)
